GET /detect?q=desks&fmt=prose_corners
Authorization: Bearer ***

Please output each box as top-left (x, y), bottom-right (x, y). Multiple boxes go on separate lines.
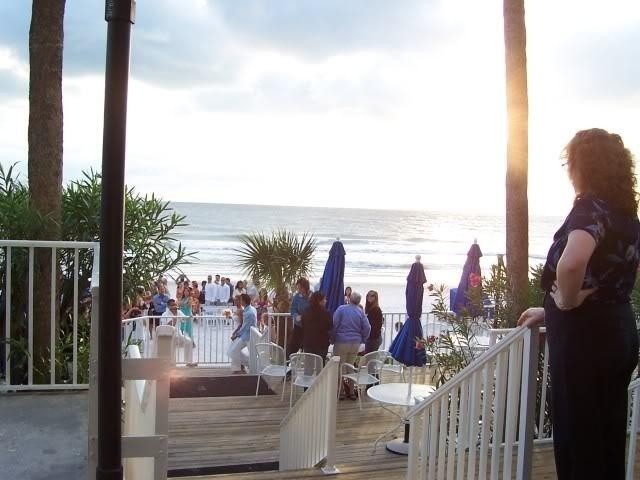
top-left (366, 383), bottom-right (436, 457)
top-left (450, 334), bottom-right (490, 359)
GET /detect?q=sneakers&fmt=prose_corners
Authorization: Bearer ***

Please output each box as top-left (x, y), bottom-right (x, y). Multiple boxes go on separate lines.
top-left (231, 366), bottom-right (246, 374)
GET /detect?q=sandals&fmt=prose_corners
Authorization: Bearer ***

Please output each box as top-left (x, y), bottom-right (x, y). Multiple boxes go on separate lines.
top-left (338, 391), bottom-right (357, 401)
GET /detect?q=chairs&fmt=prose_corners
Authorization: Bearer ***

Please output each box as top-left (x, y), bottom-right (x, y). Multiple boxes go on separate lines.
top-left (252, 348), bottom-right (406, 409)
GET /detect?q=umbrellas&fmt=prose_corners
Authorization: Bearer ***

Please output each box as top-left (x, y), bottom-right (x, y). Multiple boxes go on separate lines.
top-left (389, 254), bottom-right (430, 441)
top-left (316, 238), bottom-right (347, 346)
top-left (450, 238), bottom-right (488, 332)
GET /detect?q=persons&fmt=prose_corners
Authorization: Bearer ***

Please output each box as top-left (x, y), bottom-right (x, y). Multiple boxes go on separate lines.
top-left (395, 323), bottom-right (403, 331)
top-left (229, 294), bottom-right (249, 375)
top-left (289, 274), bottom-right (383, 400)
top-left (343, 286), bottom-right (352, 305)
top-left (122, 266), bottom-right (274, 348)
top-left (516, 127), bottom-right (640, 480)
top-left (314, 278), bottom-right (322, 293)
top-left (225, 294), bottom-right (258, 374)
top-left (158, 300), bottom-right (198, 368)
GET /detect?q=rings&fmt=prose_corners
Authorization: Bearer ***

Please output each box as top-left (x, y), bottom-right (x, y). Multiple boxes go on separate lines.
top-left (553, 289), bottom-right (558, 293)
